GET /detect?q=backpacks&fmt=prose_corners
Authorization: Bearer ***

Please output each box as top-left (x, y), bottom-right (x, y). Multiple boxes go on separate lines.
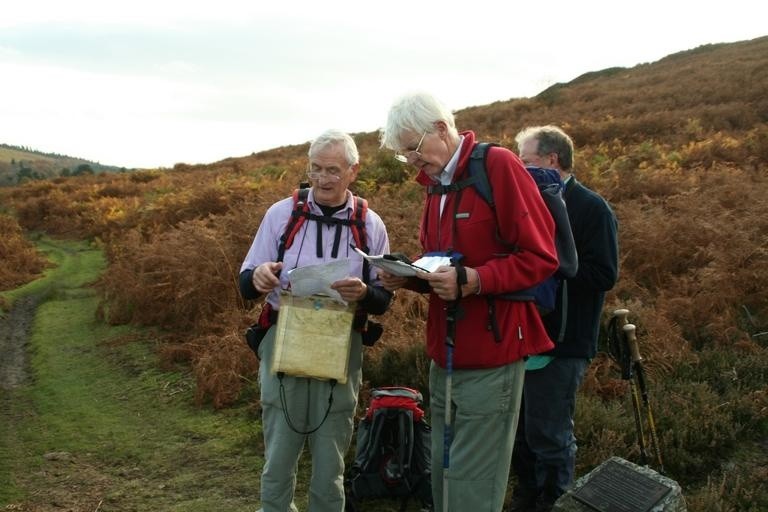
top-left (469, 134), bottom-right (578, 280)
top-left (350, 386), bottom-right (431, 500)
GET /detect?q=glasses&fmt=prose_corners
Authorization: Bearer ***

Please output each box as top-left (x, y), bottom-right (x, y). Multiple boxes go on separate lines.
top-left (395, 130), bottom-right (428, 163)
top-left (305, 163), bottom-right (351, 183)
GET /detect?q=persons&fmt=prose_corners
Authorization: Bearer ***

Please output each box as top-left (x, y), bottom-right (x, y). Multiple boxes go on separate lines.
top-left (510, 123), bottom-right (619, 512)
top-left (237, 129), bottom-right (396, 512)
top-left (378, 90), bottom-right (560, 512)
